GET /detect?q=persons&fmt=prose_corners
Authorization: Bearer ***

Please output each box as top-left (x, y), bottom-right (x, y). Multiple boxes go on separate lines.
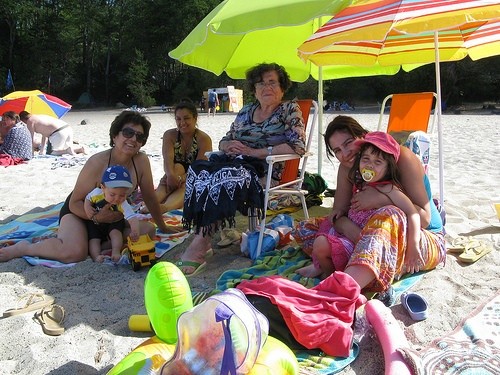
top-left (140, 97), bottom-right (213, 215)
top-left (206, 88), bottom-right (220, 117)
top-left (293, 115), bottom-right (446, 289)
top-left (0, 111), bottom-right (182, 264)
top-left (84, 165), bottom-right (140, 263)
top-left (19, 111), bottom-right (89, 158)
top-left (171, 62), bottom-right (307, 276)
top-left (0, 111), bottom-right (33, 160)
top-left (199, 95), bottom-right (206, 113)
top-left (294, 131), bottom-right (426, 279)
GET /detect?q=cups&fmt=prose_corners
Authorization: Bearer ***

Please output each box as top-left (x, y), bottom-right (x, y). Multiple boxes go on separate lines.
top-left (128, 314), bottom-right (154, 332)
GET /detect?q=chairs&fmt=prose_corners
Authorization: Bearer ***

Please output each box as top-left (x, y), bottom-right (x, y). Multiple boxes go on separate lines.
top-left (204, 99), bottom-right (319, 263)
top-left (377, 90), bottom-right (437, 136)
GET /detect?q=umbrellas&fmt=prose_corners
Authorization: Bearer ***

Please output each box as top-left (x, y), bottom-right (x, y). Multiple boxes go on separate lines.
top-left (168, 0), bottom-right (431, 175)
top-left (0, 90), bottom-right (72, 119)
top-left (297, 0), bottom-right (500, 208)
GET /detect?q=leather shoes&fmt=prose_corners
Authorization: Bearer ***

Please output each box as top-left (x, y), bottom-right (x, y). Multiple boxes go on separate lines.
top-left (217, 228), bottom-right (240, 245)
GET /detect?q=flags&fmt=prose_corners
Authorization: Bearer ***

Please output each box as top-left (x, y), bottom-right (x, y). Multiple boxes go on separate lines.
top-left (6, 71), bottom-right (13, 89)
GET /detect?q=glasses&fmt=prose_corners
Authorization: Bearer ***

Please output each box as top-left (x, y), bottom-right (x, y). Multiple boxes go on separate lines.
top-left (121, 128), bottom-right (146, 143)
top-left (254, 82), bottom-right (281, 89)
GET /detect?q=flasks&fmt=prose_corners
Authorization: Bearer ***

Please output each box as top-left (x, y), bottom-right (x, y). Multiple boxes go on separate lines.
top-left (248, 206), bottom-right (260, 232)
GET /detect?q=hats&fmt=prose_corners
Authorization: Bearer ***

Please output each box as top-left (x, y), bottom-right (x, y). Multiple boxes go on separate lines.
top-left (101, 166), bottom-right (133, 189)
top-left (355, 131), bottom-right (400, 163)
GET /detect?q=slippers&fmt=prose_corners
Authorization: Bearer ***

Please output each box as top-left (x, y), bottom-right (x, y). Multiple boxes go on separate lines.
top-left (459, 244), bottom-right (491, 262)
top-left (175, 261), bottom-right (207, 276)
top-left (175, 249), bottom-right (213, 261)
top-left (36, 304), bottom-right (64, 335)
top-left (4, 293), bottom-right (54, 317)
top-left (447, 237), bottom-right (480, 249)
top-left (401, 290), bottom-right (427, 319)
